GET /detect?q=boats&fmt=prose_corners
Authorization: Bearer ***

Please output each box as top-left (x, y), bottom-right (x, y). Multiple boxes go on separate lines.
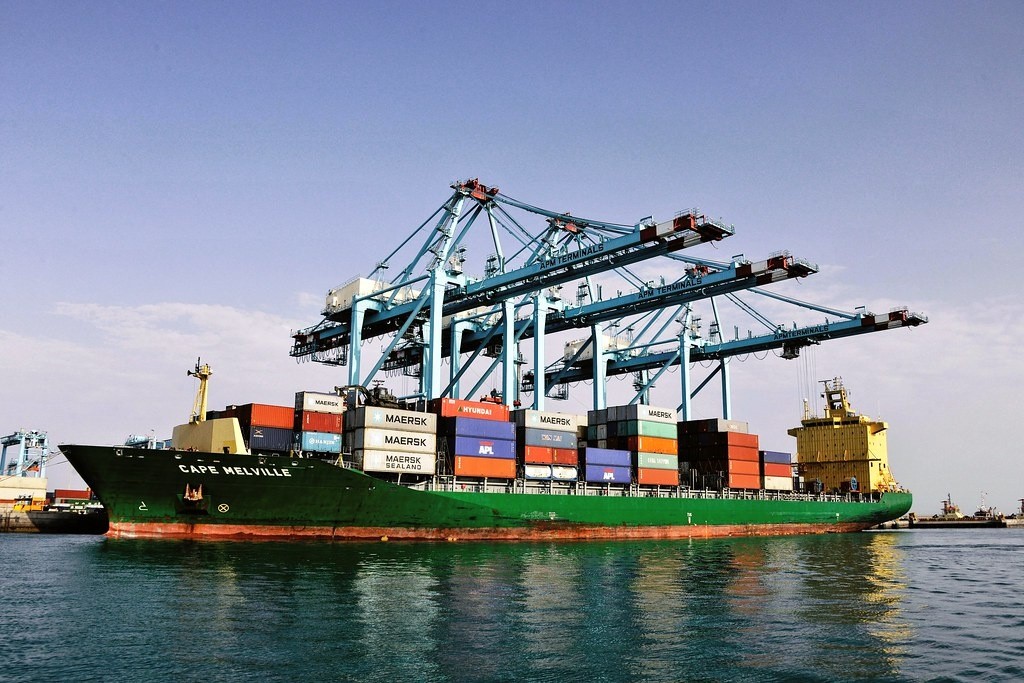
top-left (25, 506), bottom-right (109, 535)
top-left (0, 427), bottom-right (172, 532)
top-left (57, 177), bottom-right (929, 542)
